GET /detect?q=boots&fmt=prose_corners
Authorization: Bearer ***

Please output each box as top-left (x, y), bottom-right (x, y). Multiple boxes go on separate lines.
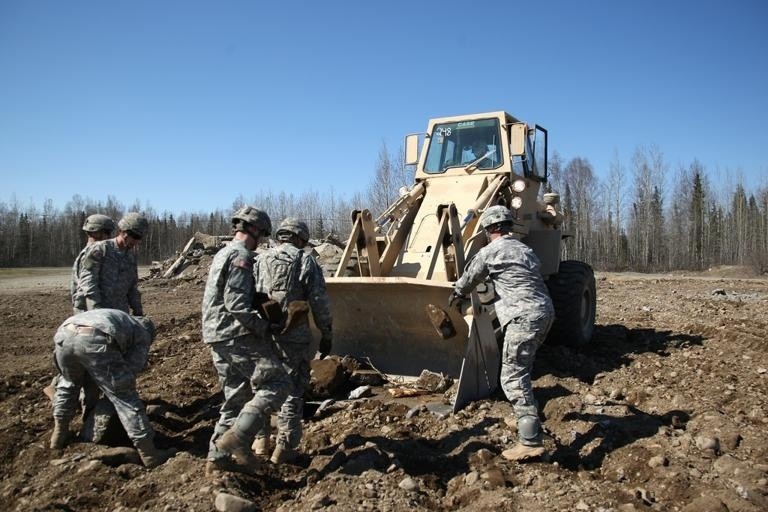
top-left (137, 437), bottom-right (177, 467)
top-left (49, 417), bottom-right (68, 449)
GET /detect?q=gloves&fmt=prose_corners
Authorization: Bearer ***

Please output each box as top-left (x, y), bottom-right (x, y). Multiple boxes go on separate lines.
top-left (319, 337), bottom-right (331, 359)
top-left (270, 309), bottom-right (288, 336)
top-left (253, 292), bottom-right (267, 307)
top-left (449, 292), bottom-right (459, 306)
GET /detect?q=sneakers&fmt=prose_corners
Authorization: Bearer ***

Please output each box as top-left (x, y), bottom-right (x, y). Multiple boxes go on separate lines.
top-left (508, 419), bottom-right (518, 429)
top-left (208, 430), bottom-right (299, 475)
top-left (502, 443), bottom-right (545, 460)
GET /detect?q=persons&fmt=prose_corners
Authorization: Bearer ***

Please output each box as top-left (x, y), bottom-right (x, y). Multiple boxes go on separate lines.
top-left (73, 211), bottom-right (149, 439)
top-left (250, 216), bottom-right (335, 466)
top-left (49, 306), bottom-right (163, 467)
top-left (40, 213), bottom-right (115, 405)
top-left (200, 205), bottom-right (293, 470)
top-left (448, 204), bottom-right (556, 462)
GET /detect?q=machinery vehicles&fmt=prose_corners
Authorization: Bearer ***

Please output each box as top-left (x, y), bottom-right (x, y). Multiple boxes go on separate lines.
top-left (307, 111), bottom-right (596, 416)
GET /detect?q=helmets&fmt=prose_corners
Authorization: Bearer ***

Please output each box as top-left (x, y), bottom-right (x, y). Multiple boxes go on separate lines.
top-left (481, 205), bottom-right (513, 229)
top-left (231, 206), bottom-right (271, 238)
top-left (118, 212), bottom-right (148, 237)
top-left (82, 214), bottom-right (114, 232)
top-left (134, 315), bottom-right (157, 343)
top-left (275, 217), bottom-right (309, 245)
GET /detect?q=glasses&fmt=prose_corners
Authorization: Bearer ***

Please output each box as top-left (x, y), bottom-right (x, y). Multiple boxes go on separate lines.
top-left (128, 231), bottom-right (141, 240)
